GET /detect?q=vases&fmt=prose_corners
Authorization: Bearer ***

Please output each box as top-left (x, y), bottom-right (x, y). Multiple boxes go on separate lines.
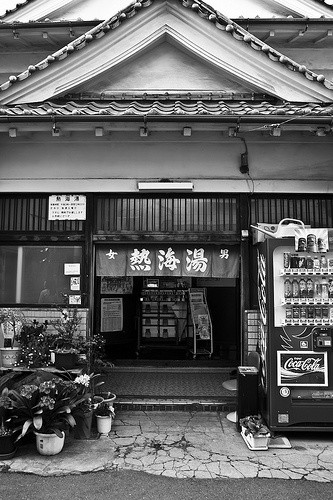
top-left (87, 396), bottom-right (104, 411)
top-left (95, 414), bottom-right (112, 434)
top-left (73, 411), bottom-right (93, 440)
top-left (55, 353), bottom-right (78, 371)
top-left (94, 391), bottom-right (116, 408)
top-left (0, 435), bottom-right (16, 454)
top-left (33, 430), bottom-right (66, 456)
top-left (0, 348), bottom-right (21, 367)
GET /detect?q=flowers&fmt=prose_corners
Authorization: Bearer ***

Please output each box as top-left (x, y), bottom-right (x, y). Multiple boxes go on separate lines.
top-left (0, 293), bottom-right (115, 445)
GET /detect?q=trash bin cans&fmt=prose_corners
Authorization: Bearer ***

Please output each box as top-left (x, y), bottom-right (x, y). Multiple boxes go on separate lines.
top-left (237, 366), bottom-right (259, 432)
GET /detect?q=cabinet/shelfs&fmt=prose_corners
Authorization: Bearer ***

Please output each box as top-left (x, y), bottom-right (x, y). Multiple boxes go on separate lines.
top-left (139, 292), bottom-right (190, 356)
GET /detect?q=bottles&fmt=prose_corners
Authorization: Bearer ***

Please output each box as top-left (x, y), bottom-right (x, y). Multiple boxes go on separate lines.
top-left (182, 291), bottom-right (186, 301)
top-left (163, 318), bottom-right (168, 326)
top-left (163, 328), bottom-right (169, 338)
top-left (284, 277), bottom-right (333, 299)
top-left (307, 234), bottom-right (317, 253)
top-left (145, 329), bottom-right (151, 338)
top-left (145, 304), bottom-right (151, 313)
top-left (162, 304), bottom-right (167, 313)
top-left (318, 238), bottom-right (325, 249)
top-left (284, 250), bottom-right (327, 269)
top-left (145, 318), bottom-right (151, 325)
top-left (299, 237), bottom-right (306, 251)
top-left (286, 304), bottom-right (329, 322)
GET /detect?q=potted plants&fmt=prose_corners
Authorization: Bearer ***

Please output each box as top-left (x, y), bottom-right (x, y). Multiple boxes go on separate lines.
top-left (239, 414), bottom-right (271, 451)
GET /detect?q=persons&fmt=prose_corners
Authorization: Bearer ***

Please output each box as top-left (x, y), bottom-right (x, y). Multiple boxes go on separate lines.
top-left (38, 277), bottom-right (65, 304)
